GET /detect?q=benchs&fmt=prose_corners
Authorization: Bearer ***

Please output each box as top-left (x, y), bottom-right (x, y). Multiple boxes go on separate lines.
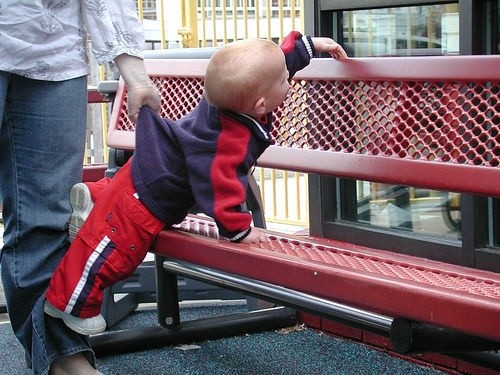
top-left (82, 57), bottom-right (500, 374)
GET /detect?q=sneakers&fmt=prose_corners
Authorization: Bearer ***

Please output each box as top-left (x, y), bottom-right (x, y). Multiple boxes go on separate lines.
top-left (41, 301), bottom-right (106, 338)
top-left (67, 182), bottom-right (93, 245)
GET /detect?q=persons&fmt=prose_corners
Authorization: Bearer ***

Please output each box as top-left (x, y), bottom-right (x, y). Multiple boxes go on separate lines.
top-left (1, 0), bottom-right (162, 375)
top-left (43, 30), bottom-right (348, 336)
